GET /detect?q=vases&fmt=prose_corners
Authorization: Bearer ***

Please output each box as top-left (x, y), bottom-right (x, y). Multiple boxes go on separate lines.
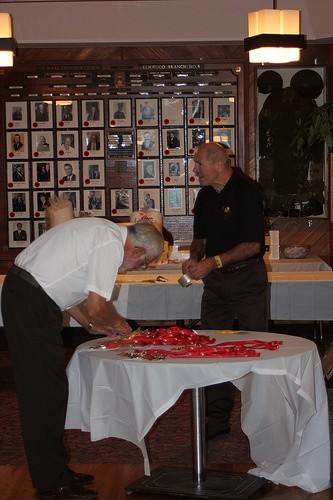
top-left (254, 65), bottom-right (330, 220)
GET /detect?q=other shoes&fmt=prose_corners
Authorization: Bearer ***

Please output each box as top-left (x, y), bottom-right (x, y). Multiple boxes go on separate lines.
top-left (206, 419), bottom-right (232, 438)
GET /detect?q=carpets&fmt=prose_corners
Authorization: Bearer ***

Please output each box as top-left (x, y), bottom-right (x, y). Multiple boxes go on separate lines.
top-left (0, 349), bottom-right (260, 465)
top-left (64, 329), bottom-right (332, 500)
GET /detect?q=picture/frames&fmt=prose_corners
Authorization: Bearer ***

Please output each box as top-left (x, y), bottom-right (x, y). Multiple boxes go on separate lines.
top-left (109, 187), bottom-right (135, 218)
top-left (3, 98), bottom-right (107, 249)
top-left (107, 97), bottom-right (133, 128)
top-left (134, 95), bottom-right (236, 216)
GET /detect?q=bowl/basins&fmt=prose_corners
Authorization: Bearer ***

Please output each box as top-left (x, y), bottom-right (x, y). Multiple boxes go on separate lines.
top-left (281, 245), bottom-right (311, 259)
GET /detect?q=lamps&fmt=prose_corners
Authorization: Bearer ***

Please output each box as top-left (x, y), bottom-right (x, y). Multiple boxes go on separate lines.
top-left (243, 0), bottom-right (306, 64)
top-left (0, 12), bottom-right (16, 67)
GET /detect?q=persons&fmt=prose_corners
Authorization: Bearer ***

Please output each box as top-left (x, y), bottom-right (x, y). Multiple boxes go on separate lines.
top-left (39, 223), bottom-right (49, 236)
top-left (116, 193), bottom-right (129, 209)
top-left (0, 217), bottom-right (165, 500)
top-left (113, 101), bottom-right (154, 120)
top-left (138, 130), bottom-right (183, 210)
top-left (12, 191), bottom-right (101, 213)
top-left (181, 142), bottom-right (269, 441)
top-left (189, 100), bottom-right (235, 150)
top-left (12, 222), bottom-right (27, 241)
top-left (12, 163), bottom-right (100, 182)
top-left (12, 103), bottom-right (100, 121)
top-left (11, 132), bottom-right (100, 154)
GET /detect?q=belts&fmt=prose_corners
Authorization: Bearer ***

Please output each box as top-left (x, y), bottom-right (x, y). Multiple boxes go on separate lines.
top-left (10, 264), bottom-right (45, 291)
top-left (220, 263), bottom-right (249, 270)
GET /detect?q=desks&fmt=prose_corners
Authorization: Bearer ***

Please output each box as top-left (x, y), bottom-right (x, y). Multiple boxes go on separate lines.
top-left (0, 253), bottom-right (333, 345)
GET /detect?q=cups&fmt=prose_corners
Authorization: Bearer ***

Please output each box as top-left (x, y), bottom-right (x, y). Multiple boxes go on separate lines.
top-left (178, 272), bottom-right (193, 288)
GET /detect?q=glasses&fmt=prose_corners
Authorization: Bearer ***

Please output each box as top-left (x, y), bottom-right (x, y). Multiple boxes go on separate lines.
top-left (140, 215), bottom-right (154, 224)
top-left (140, 254), bottom-right (147, 270)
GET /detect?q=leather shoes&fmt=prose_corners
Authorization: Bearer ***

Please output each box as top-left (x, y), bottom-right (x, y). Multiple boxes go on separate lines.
top-left (68, 467), bottom-right (94, 486)
top-left (36, 481), bottom-right (96, 500)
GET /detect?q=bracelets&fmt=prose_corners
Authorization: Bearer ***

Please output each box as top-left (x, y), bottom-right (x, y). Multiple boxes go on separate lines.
top-left (214, 255), bottom-right (223, 268)
top-left (86, 321), bottom-right (94, 331)
